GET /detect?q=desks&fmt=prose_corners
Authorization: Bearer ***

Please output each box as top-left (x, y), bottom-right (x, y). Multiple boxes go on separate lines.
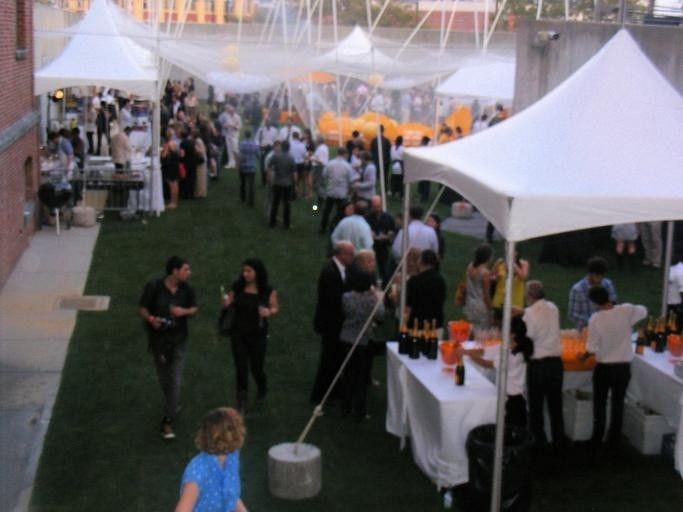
top-left (41, 108), bottom-right (167, 213)
top-left (383, 329), bottom-right (682, 511)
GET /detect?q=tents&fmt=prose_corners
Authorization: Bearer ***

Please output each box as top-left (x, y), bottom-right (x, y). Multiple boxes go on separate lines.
top-left (397, 26), bottom-right (679, 337)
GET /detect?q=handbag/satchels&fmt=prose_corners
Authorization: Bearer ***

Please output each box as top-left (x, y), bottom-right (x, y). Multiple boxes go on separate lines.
top-left (219, 303), bottom-right (235, 334)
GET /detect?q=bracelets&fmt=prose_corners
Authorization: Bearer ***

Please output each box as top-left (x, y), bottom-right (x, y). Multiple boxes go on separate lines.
top-left (267, 307), bottom-right (273, 315)
top-left (145, 315), bottom-right (154, 323)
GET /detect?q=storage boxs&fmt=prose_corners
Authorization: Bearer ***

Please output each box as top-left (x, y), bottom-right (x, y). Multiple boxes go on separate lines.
top-left (624, 396), bottom-right (676, 455)
top-left (661, 432), bottom-right (676, 458)
top-left (562, 390), bottom-right (611, 441)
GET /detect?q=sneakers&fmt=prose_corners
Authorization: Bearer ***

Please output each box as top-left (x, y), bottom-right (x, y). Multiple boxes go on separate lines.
top-left (161, 423), bottom-right (175, 440)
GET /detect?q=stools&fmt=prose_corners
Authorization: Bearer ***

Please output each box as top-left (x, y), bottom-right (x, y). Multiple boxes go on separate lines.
top-left (34, 186), bottom-right (70, 236)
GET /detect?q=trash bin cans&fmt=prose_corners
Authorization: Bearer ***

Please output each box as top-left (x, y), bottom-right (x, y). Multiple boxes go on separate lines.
top-left (466, 424), bottom-right (535, 512)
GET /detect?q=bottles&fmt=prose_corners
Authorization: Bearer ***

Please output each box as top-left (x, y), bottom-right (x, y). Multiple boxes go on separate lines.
top-left (454, 356), bottom-right (465, 386)
top-left (634, 310), bottom-right (677, 354)
top-left (153, 318), bottom-right (170, 327)
top-left (398, 316), bottom-right (439, 359)
top-left (219, 285), bottom-right (229, 308)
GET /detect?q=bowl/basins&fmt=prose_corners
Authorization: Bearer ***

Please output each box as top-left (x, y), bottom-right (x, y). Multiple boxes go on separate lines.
top-left (448, 321), bottom-right (471, 341)
top-left (440, 343), bottom-right (462, 365)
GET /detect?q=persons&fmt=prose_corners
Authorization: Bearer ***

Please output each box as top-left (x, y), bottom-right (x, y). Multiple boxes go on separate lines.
top-left (668, 255), bottom-right (681, 335)
top-left (311, 102), bottom-right (510, 306)
top-left (464, 244), bottom-right (529, 323)
top-left (51, 91), bottom-right (152, 201)
top-left (311, 242), bottom-right (447, 403)
top-left (157, 78), bottom-right (311, 231)
top-left (567, 285), bottom-right (649, 449)
top-left (136, 255), bottom-right (198, 440)
top-left (470, 280), bottom-right (563, 456)
top-left (567, 257), bottom-right (616, 327)
top-left (172, 404), bottom-right (252, 512)
top-left (220, 256), bottom-right (281, 418)
top-left (538, 221), bottom-right (662, 270)
top-left (338, 255), bottom-right (385, 416)
